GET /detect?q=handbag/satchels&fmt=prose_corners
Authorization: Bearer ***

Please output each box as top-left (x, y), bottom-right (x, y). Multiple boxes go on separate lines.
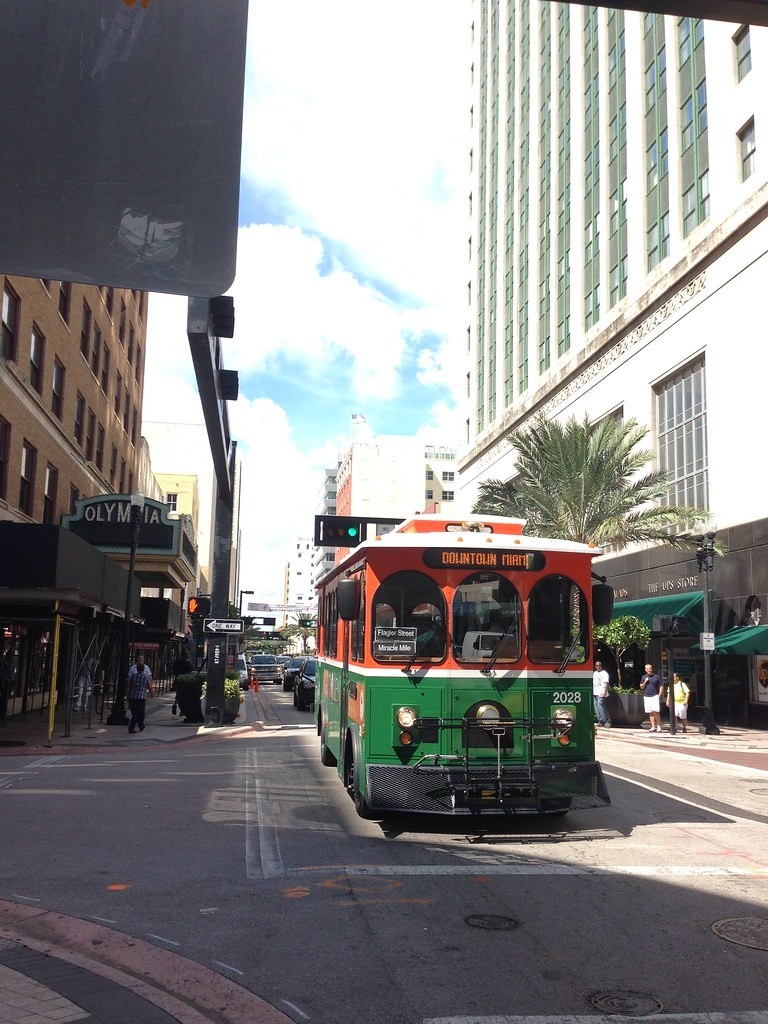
top-left (86, 686), bottom-right (92, 696)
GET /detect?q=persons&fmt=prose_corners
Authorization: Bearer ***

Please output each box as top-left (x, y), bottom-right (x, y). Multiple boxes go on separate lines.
top-left (467, 608), bottom-right (506, 652)
top-left (126, 655), bottom-right (154, 734)
top-left (73, 650), bottom-right (94, 711)
top-left (640, 664), bottom-right (664, 732)
top-left (172, 650), bottom-right (193, 716)
top-left (666, 672), bottom-right (690, 733)
top-left (593, 661), bottom-right (611, 728)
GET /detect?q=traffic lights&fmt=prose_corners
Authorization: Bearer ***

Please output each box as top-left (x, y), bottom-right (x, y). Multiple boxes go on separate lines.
top-left (299, 619), bottom-right (311, 627)
top-left (652, 617), bottom-right (669, 632)
top-left (671, 616), bottom-right (689, 632)
top-left (314, 515), bottom-right (369, 547)
top-left (188, 597), bottom-right (210, 615)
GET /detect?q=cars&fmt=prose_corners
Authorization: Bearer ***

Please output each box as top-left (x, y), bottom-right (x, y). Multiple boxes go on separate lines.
top-left (283, 656), bottom-right (316, 692)
top-left (293, 657), bottom-right (319, 712)
top-left (276, 655), bottom-right (293, 667)
top-left (247, 654), bottom-right (284, 685)
top-left (238, 654), bottom-right (250, 691)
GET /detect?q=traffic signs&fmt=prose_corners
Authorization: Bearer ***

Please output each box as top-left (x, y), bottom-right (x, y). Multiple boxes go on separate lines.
top-left (203, 618), bottom-right (245, 635)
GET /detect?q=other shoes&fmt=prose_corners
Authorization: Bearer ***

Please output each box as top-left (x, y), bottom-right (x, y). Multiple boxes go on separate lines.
top-left (669, 727), bottom-right (677, 732)
top-left (683, 728), bottom-right (686, 733)
top-left (657, 725), bottom-right (662, 732)
top-left (172, 704), bottom-right (177, 715)
top-left (84, 707), bottom-right (87, 711)
top-left (649, 727), bottom-right (657, 732)
top-left (74, 707), bottom-right (79, 711)
top-left (596, 723), bottom-right (604, 727)
top-left (603, 722), bottom-right (611, 728)
top-left (179, 712), bottom-right (184, 716)
top-left (139, 726), bottom-right (145, 731)
top-left (129, 730), bottom-right (137, 734)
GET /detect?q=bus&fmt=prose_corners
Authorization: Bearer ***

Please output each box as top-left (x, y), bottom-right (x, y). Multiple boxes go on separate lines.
top-left (313, 514), bottom-right (612, 821)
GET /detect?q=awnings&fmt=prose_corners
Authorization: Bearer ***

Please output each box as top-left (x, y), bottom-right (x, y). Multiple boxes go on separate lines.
top-left (609, 591), bottom-right (713, 635)
top-left (690, 625), bottom-right (768, 655)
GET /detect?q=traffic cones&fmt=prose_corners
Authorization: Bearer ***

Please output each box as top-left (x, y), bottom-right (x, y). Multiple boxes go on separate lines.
top-left (254, 679), bottom-right (260, 692)
top-left (250, 675), bottom-right (255, 689)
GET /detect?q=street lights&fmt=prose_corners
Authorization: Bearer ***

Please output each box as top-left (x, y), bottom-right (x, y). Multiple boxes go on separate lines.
top-left (106, 489), bottom-right (146, 726)
top-left (239, 590), bottom-right (254, 618)
top-left (696, 532), bottom-right (721, 736)
